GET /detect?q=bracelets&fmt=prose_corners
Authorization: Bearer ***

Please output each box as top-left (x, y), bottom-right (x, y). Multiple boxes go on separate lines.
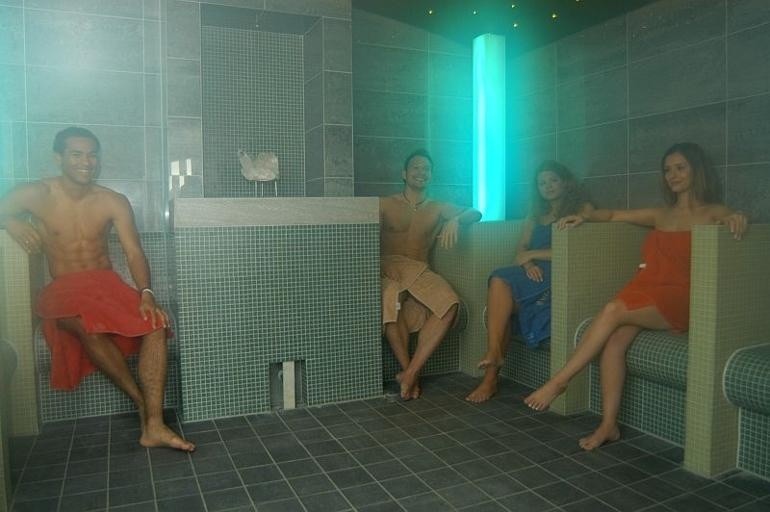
top-left (140, 287), bottom-right (155, 296)
top-left (452, 214), bottom-right (462, 226)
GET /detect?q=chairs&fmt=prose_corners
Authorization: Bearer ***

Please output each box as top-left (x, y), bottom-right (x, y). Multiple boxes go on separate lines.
top-left (1, 214), bottom-right (179, 437)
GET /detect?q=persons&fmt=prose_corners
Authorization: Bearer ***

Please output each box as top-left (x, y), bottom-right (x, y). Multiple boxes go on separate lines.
top-left (523, 143), bottom-right (749, 452)
top-left (379, 149), bottom-right (482, 402)
top-left (465, 159), bottom-right (598, 403)
top-left (0, 127), bottom-right (196, 453)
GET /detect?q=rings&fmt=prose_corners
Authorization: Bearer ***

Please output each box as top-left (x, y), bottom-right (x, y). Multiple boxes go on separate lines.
top-left (24, 240), bottom-right (30, 244)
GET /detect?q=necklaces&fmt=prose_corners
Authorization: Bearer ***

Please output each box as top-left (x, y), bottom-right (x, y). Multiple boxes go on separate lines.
top-left (403, 190), bottom-right (427, 212)
